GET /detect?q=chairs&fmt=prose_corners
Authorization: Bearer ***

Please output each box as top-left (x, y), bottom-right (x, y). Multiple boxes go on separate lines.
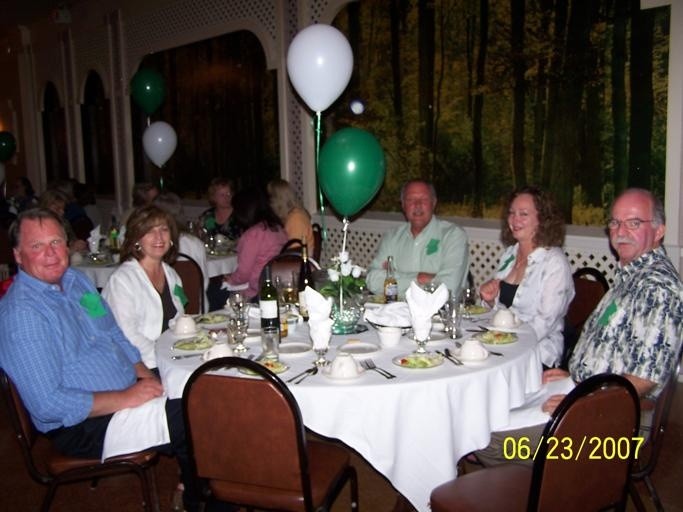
top-left (460, 271), bottom-right (475, 301)
top-left (564, 267), bottom-right (610, 334)
top-left (72, 256), bottom-right (240, 286)
top-left (171, 252), bottom-right (203, 318)
top-left (259, 253), bottom-right (322, 298)
top-left (312, 222), bottom-right (323, 264)
top-left (0, 371), bottom-right (161, 511)
top-left (629, 366), bottom-right (673, 512)
top-left (281, 238), bottom-right (309, 255)
top-left (431, 373), bottom-right (642, 512)
top-left (178, 356), bottom-right (361, 512)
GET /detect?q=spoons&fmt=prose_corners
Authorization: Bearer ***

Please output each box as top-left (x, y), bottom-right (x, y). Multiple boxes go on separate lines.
top-left (295, 368), bottom-right (318, 385)
top-left (445, 348), bottom-right (464, 366)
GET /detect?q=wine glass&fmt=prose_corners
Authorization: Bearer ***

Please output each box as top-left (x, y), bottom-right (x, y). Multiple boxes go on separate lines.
top-left (229, 292), bottom-right (250, 335)
top-left (225, 312), bottom-right (249, 353)
top-left (461, 288), bottom-right (479, 320)
top-left (436, 301), bottom-right (453, 335)
top-left (411, 326), bottom-right (432, 352)
top-left (356, 287), bottom-right (368, 317)
top-left (308, 336), bottom-right (334, 365)
top-left (424, 277), bottom-right (440, 293)
top-left (445, 298), bottom-right (464, 340)
top-left (280, 280), bottom-right (300, 317)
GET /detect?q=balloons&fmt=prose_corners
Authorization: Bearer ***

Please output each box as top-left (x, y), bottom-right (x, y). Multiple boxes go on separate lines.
top-left (142, 121), bottom-right (178, 168)
top-left (285, 22), bottom-right (356, 114)
top-left (317, 127), bottom-right (387, 218)
top-left (0, 131), bottom-right (17, 164)
top-left (130, 68), bottom-right (165, 115)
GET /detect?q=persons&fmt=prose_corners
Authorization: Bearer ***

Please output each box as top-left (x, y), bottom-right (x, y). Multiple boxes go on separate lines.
top-left (2, 176), bottom-right (246, 512)
top-left (478, 185), bottom-right (576, 373)
top-left (365, 177), bottom-right (471, 303)
top-left (208, 186), bottom-right (289, 312)
top-left (462, 185), bottom-right (683, 468)
top-left (266, 177), bottom-right (316, 259)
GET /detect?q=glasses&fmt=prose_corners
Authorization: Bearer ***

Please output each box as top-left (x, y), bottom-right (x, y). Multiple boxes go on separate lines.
top-left (607, 217), bottom-right (658, 230)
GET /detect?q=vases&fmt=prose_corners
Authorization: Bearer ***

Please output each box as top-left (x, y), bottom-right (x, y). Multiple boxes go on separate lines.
top-left (329, 292), bottom-right (361, 335)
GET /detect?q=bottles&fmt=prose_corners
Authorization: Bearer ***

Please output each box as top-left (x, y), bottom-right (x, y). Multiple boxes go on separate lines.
top-left (297, 237), bottom-right (315, 321)
top-left (259, 264), bottom-right (281, 334)
top-left (385, 256), bottom-right (399, 304)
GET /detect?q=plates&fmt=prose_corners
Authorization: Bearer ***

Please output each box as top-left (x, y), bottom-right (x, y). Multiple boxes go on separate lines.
top-left (473, 330), bottom-right (519, 347)
top-left (237, 362), bottom-right (290, 377)
top-left (198, 321), bottom-right (230, 331)
top-left (449, 349), bottom-right (490, 366)
top-left (243, 328), bottom-right (261, 341)
top-left (391, 354), bottom-right (444, 368)
top-left (172, 336), bottom-right (211, 352)
top-left (279, 342), bottom-right (312, 357)
top-left (338, 342), bottom-right (378, 356)
top-left (463, 310), bottom-right (495, 319)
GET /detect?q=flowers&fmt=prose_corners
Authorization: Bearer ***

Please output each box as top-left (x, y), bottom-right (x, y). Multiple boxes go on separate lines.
top-left (320, 251), bottom-right (369, 292)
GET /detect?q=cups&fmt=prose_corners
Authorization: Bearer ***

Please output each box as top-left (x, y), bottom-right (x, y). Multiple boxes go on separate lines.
top-left (169, 315), bottom-right (196, 333)
top-left (491, 308), bottom-right (521, 328)
top-left (202, 342), bottom-right (232, 362)
top-left (329, 353), bottom-right (359, 378)
top-left (460, 337), bottom-right (489, 361)
top-left (260, 326), bottom-right (280, 365)
top-left (376, 327), bottom-right (403, 347)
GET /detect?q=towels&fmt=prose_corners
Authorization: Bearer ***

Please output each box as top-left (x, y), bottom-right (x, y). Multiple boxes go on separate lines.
top-left (305, 285), bottom-right (334, 349)
top-left (405, 282), bottom-right (448, 342)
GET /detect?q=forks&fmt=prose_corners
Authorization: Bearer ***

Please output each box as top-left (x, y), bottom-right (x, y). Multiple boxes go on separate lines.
top-left (360, 361), bottom-right (390, 379)
top-left (478, 325), bottom-right (516, 336)
top-left (365, 358), bottom-right (396, 378)
top-left (172, 352), bottom-right (204, 360)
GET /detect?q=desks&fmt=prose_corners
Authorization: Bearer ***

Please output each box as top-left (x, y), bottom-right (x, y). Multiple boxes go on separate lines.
top-left (154, 295), bottom-right (543, 511)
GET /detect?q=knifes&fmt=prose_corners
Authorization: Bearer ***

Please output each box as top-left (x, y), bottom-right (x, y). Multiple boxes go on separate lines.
top-left (465, 329), bottom-right (488, 332)
top-left (287, 368), bottom-right (312, 384)
top-left (435, 350), bottom-right (459, 368)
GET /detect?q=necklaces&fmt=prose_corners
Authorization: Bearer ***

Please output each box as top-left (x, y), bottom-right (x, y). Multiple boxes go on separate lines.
top-left (515, 251), bottom-right (526, 268)
